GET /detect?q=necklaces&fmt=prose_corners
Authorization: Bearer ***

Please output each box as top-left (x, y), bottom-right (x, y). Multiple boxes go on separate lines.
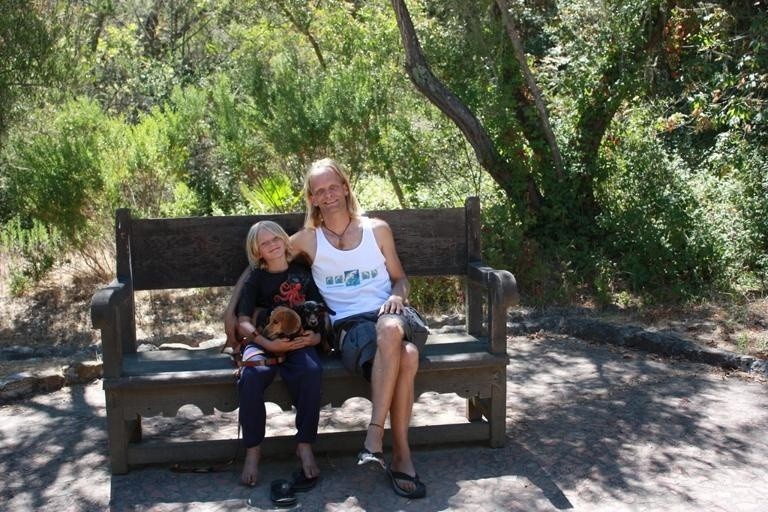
top-left (323, 219), bottom-right (353, 250)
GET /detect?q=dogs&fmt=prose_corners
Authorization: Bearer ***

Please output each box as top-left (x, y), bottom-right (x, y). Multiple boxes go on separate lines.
top-left (251, 300), bottom-right (338, 364)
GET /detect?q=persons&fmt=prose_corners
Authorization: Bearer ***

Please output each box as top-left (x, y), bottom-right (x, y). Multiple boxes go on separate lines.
top-left (236, 221), bottom-right (334, 488)
top-left (224, 157), bottom-right (432, 499)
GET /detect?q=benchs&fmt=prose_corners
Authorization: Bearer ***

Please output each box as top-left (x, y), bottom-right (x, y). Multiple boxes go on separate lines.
top-left (90, 197), bottom-right (522, 476)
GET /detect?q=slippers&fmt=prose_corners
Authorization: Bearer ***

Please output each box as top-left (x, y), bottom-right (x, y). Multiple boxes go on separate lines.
top-left (387, 462), bottom-right (426, 498)
top-left (270, 479), bottom-right (296, 505)
top-left (353, 449), bottom-right (387, 471)
top-left (292, 470), bottom-right (316, 492)
